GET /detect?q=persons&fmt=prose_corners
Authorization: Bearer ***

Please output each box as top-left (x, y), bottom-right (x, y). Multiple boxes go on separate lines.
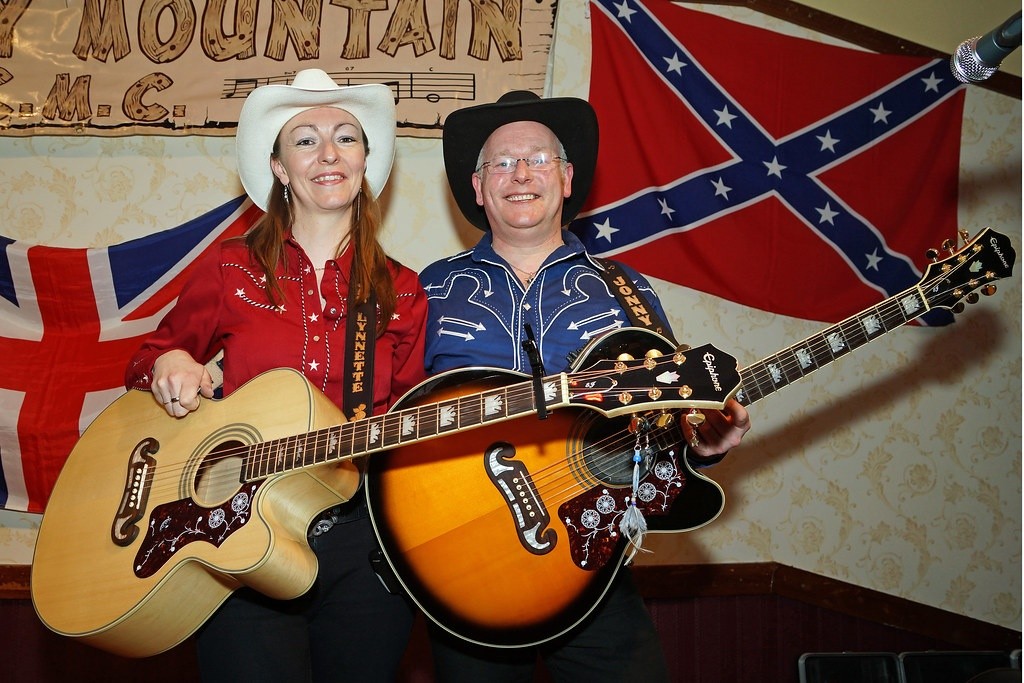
top-left (419, 89), bottom-right (753, 682)
top-left (125, 67), bottom-right (426, 683)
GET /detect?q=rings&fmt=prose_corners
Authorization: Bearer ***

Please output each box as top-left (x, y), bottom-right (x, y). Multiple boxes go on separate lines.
top-left (163, 396), bottom-right (179, 405)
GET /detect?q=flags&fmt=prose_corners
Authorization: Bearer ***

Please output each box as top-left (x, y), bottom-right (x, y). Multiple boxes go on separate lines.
top-left (0, 192), bottom-right (269, 515)
top-left (544, 0), bottom-right (965, 329)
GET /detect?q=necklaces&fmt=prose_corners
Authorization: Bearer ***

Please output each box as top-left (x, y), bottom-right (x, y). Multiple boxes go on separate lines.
top-left (491, 243), bottom-right (566, 283)
top-left (313, 268), bottom-right (326, 271)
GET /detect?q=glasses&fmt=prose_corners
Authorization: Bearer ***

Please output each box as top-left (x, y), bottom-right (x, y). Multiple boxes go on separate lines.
top-left (476, 152), bottom-right (569, 174)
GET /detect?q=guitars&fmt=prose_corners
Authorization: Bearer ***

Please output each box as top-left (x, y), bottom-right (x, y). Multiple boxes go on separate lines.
top-left (28, 342), bottom-right (744, 662)
top-left (362, 225), bottom-right (1018, 650)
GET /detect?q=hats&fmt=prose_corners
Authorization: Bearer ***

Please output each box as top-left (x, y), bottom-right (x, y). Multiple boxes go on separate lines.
top-left (443, 91), bottom-right (599, 233)
top-left (235, 69), bottom-right (397, 214)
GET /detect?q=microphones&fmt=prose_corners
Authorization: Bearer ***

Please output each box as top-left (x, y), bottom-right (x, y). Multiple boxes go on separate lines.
top-left (950, 9), bottom-right (1022, 84)
